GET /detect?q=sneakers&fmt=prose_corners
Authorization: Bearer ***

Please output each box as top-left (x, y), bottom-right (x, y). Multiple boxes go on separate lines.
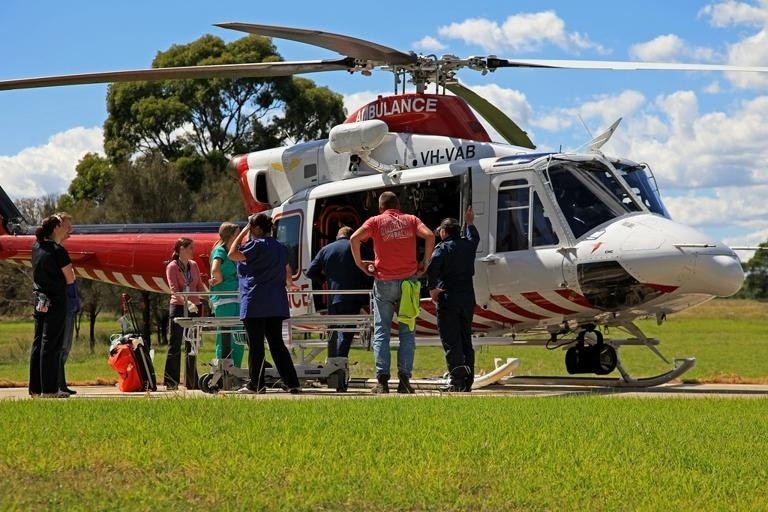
top-left (40, 389), bottom-right (71, 399)
top-left (185, 382), bottom-right (200, 391)
top-left (396, 373), bottom-right (416, 393)
top-left (59, 382), bottom-right (77, 395)
top-left (166, 381), bottom-right (179, 391)
top-left (439, 378), bottom-right (473, 393)
top-left (30, 393), bottom-right (43, 400)
top-left (370, 373), bottom-right (390, 394)
top-left (282, 381), bottom-right (302, 395)
top-left (236, 383), bottom-right (267, 395)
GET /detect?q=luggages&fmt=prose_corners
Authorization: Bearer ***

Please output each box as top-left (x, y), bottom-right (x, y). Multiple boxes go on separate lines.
top-left (107, 293), bottom-right (157, 392)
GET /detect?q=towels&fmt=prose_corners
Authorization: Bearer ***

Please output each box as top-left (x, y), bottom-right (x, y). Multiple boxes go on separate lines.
top-left (396, 281), bottom-right (420, 331)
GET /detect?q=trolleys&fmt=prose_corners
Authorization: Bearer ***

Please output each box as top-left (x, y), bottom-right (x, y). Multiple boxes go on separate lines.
top-left (172, 290), bottom-right (374, 392)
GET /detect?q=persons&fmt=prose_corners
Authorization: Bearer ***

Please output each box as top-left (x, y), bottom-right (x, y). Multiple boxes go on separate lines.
top-left (304, 225), bottom-right (373, 391)
top-left (163, 237), bottom-right (209, 391)
top-left (208, 222), bottom-right (241, 367)
top-left (227, 213), bottom-right (300, 394)
top-left (29, 216), bottom-right (74, 397)
top-left (350, 190), bottom-right (435, 394)
top-left (51, 212), bottom-right (82, 394)
top-left (427, 205), bottom-right (480, 391)
